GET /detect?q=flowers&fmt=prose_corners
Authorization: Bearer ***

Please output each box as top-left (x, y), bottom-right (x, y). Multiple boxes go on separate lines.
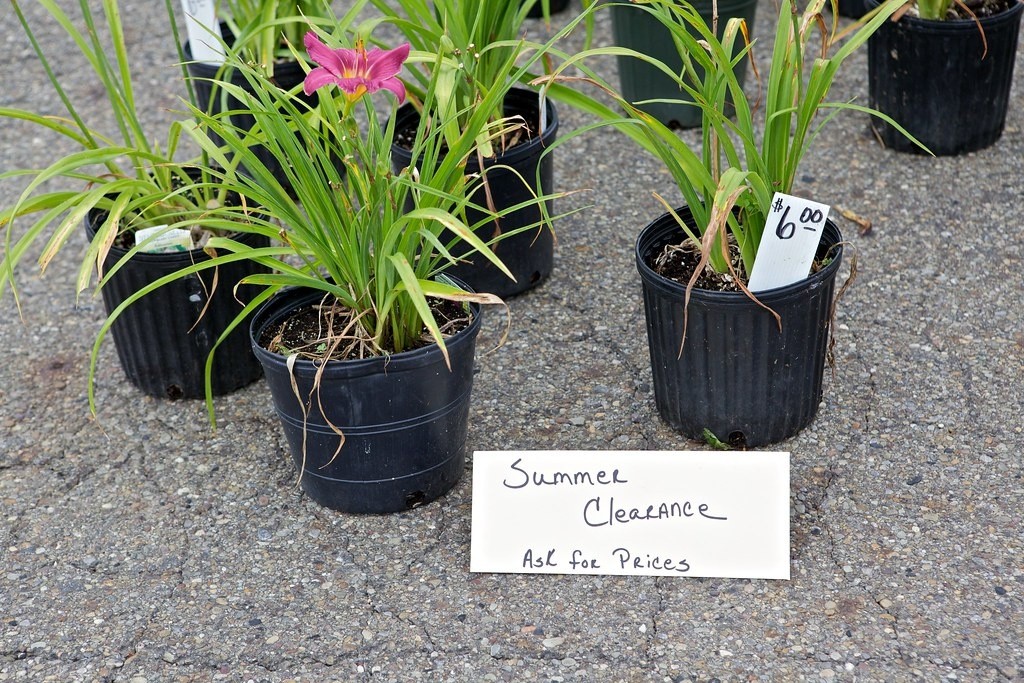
top-left (83, 0), bottom-right (563, 411)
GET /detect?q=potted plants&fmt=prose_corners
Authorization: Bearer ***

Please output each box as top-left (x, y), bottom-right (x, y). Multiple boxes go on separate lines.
top-left (0, 0), bottom-right (1024, 449)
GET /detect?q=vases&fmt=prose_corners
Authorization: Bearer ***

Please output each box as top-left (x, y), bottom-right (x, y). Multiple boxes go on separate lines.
top-left (249, 278), bottom-right (481, 515)
